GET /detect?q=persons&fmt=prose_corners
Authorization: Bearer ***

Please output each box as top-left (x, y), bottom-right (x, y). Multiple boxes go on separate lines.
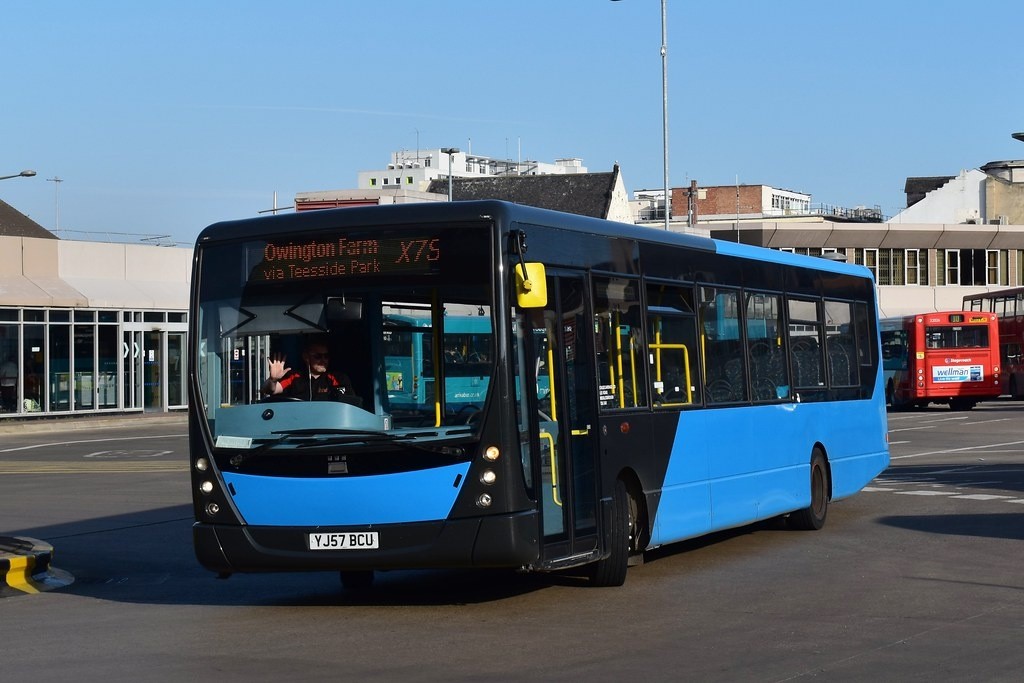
top-left (263, 339), bottom-right (349, 397)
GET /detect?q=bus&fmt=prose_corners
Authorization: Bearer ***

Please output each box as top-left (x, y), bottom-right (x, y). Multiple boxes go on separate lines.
top-left (839, 311), bottom-right (1003, 413)
top-left (185, 199), bottom-right (890, 587)
top-left (961, 286), bottom-right (1024, 401)
top-left (376, 288), bottom-right (772, 430)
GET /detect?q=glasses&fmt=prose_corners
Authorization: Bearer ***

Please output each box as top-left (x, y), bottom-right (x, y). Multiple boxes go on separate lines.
top-left (307, 351), bottom-right (331, 362)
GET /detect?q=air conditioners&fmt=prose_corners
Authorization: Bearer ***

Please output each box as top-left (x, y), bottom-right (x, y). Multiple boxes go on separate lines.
top-left (988, 219), bottom-right (1002, 225)
top-left (966, 218), bottom-right (983, 225)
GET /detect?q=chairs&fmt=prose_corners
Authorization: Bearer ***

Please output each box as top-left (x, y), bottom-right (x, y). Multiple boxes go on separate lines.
top-left (598, 333), bottom-right (864, 407)
